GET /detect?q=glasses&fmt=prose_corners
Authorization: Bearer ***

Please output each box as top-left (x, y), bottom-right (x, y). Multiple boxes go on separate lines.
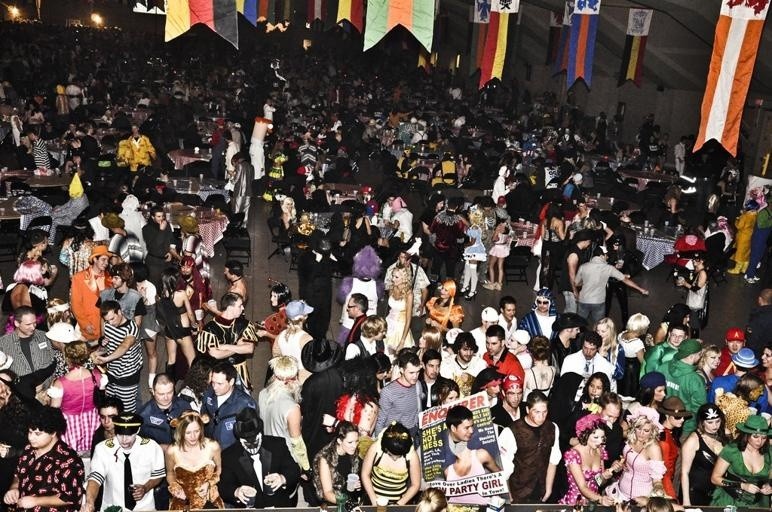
top-left (164, 409), bottom-right (172, 423)
top-left (214, 410), bottom-right (219, 425)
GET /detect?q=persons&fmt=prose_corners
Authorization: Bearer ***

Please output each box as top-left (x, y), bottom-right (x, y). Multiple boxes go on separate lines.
top-left (0, 18), bottom-right (771, 512)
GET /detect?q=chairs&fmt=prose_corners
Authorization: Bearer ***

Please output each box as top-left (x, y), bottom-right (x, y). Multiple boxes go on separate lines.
top-left (0, 64), bottom-right (737, 286)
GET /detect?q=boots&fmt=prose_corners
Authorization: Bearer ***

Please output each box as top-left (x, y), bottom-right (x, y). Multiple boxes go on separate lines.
top-left (727, 262), bottom-right (749, 274)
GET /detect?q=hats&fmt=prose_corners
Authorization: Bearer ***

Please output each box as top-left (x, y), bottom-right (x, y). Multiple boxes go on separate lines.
top-left (726, 327), bottom-right (745, 341)
top-left (513, 330), bottom-right (530, 344)
top-left (286, 301), bottom-right (314, 320)
top-left (552, 312), bottom-right (587, 331)
top-left (233, 408), bottom-right (263, 440)
top-left (45, 322), bottom-right (80, 343)
top-left (732, 348), bottom-right (759, 369)
top-left (112, 412), bottom-right (144, 435)
top-left (674, 339), bottom-right (701, 360)
top-left (301, 336), bottom-right (343, 373)
top-left (641, 372), bottom-right (772, 435)
top-left (482, 307), bottom-right (498, 321)
top-left (71, 213), bottom-right (126, 263)
top-left (502, 374), bottom-right (523, 391)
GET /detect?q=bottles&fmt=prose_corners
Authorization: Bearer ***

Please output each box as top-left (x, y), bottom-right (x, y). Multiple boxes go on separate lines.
top-left (572, 495), bottom-right (585, 512)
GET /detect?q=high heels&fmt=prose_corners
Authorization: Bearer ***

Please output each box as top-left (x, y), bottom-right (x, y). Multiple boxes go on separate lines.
top-left (458, 287), bottom-right (469, 295)
top-left (465, 289), bottom-right (479, 301)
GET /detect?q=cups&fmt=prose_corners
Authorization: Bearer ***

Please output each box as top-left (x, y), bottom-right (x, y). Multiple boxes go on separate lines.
top-left (208, 299), bottom-right (216, 308)
top-left (643, 220), bottom-right (683, 237)
top-left (346, 473), bottom-right (360, 493)
top-left (617, 256), bottom-right (625, 267)
top-left (264, 474), bottom-right (275, 496)
top-left (677, 276), bottom-right (683, 287)
top-left (158, 144), bottom-right (219, 224)
top-left (169, 244), bottom-right (177, 250)
top-left (587, 501), bottom-right (597, 512)
top-left (243, 488), bottom-right (257, 507)
top-left (376, 496), bottom-right (389, 511)
top-left (582, 192), bottom-right (615, 206)
top-left (128, 483), bottom-right (141, 501)
top-left (335, 493), bottom-right (348, 512)
top-left (0, 164), bottom-right (70, 219)
top-left (748, 406), bottom-right (757, 414)
top-left (519, 217), bottom-right (538, 239)
top-left (760, 412), bottom-right (770, 419)
top-left (195, 309), bottom-right (204, 321)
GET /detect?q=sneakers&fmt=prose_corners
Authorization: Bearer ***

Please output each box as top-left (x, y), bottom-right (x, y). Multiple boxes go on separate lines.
top-left (482, 279), bottom-right (502, 290)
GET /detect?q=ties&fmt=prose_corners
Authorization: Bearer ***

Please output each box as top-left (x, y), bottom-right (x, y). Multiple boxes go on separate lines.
top-left (251, 454), bottom-right (263, 492)
top-left (123, 452), bottom-right (135, 509)
